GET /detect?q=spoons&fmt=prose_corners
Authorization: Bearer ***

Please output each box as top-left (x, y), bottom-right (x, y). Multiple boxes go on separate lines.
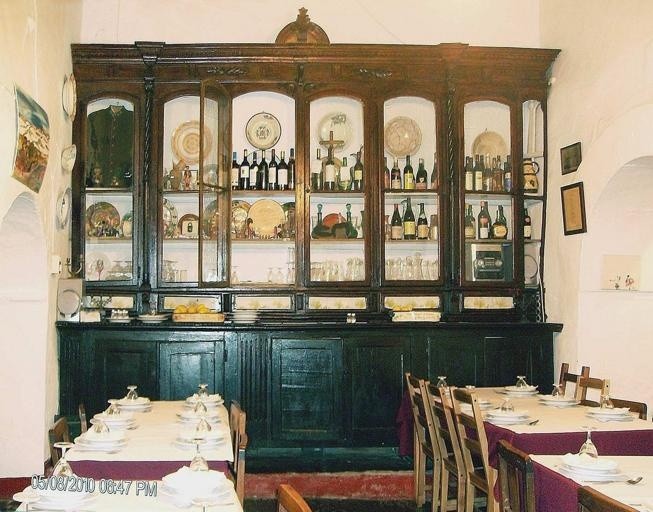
top-left (595, 476), bottom-right (644, 485)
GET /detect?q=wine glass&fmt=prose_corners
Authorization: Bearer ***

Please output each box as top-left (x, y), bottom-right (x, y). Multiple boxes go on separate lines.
top-left (74, 408), bottom-right (138, 448)
top-left (577, 426), bottom-right (598, 467)
top-left (107, 398), bottom-right (121, 418)
top-left (94, 258), bottom-right (177, 281)
top-left (52, 440), bottom-right (77, 484)
top-left (197, 383), bottom-right (210, 399)
top-left (192, 439), bottom-right (212, 477)
top-left (263, 259), bottom-right (339, 284)
top-left (94, 417), bottom-right (112, 436)
top-left (437, 375), bottom-right (447, 390)
top-left (206, 263), bottom-right (239, 284)
top-left (517, 374), bottom-right (527, 390)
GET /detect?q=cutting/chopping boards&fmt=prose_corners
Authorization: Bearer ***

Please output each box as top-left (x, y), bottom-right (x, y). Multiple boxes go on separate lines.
top-left (171, 313), bottom-right (226, 323)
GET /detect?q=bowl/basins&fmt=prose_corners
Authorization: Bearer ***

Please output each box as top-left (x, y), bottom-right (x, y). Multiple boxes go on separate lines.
top-left (60, 143), bottom-right (76, 172)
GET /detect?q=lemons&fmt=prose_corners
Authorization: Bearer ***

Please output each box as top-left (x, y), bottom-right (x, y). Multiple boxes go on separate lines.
top-left (174, 303), bottom-right (211, 313)
top-left (392, 306), bottom-right (413, 311)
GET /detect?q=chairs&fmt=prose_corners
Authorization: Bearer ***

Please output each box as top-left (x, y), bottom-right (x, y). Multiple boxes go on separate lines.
top-left (8, 393), bottom-right (254, 512)
top-left (272, 481), bottom-right (312, 511)
top-left (403, 363), bottom-right (653, 512)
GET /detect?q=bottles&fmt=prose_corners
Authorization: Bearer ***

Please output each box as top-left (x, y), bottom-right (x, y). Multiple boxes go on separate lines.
top-left (524, 207), bottom-right (532, 238)
top-left (522, 157), bottom-right (540, 193)
top-left (230, 148), bottom-right (512, 192)
top-left (311, 196), bottom-right (507, 240)
top-left (345, 251), bottom-right (440, 281)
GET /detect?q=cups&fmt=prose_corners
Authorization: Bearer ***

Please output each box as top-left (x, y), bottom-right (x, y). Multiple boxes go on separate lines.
top-left (180, 270), bottom-right (187, 282)
top-left (125, 385), bottom-right (139, 402)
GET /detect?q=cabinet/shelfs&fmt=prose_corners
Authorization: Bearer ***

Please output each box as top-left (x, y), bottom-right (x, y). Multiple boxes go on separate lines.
top-left (67, 7), bottom-right (563, 323)
top-left (81, 328), bottom-right (238, 432)
top-left (255, 329), bottom-right (423, 454)
top-left (428, 325), bottom-right (554, 392)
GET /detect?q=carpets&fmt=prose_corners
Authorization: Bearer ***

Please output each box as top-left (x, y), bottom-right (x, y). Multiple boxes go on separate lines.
top-left (241, 470), bottom-right (491, 504)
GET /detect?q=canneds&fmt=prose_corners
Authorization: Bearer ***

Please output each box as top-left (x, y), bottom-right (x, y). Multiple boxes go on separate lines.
top-left (430, 215), bottom-right (438, 240)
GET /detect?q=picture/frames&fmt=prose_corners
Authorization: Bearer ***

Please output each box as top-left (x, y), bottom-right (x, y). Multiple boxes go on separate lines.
top-left (558, 141), bottom-right (582, 176)
top-left (558, 181), bottom-right (589, 235)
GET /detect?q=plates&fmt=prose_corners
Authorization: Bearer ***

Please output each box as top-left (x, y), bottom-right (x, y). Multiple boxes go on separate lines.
top-left (559, 454), bottom-right (624, 480)
top-left (315, 112), bottom-right (354, 152)
top-left (136, 313), bottom-right (167, 323)
top-left (62, 72), bottom-right (78, 123)
top-left (384, 116), bottom-right (423, 158)
top-left (227, 310), bottom-right (261, 324)
top-left (105, 317), bottom-right (135, 323)
top-left (472, 130), bottom-right (507, 166)
top-left (87, 198), bottom-right (296, 240)
top-left (12, 474), bottom-right (96, 511)
top-left (171, 398), bottom-right (226, 446)
top-left (55, 186), bottom-right (73, 230)
top-left (106, 397), bottom-right (152, 409)
top-left (432, 382), bottom-right (636, 426)
top-left (244, 111), bottom-right (282, 150)
top-left (172, 120), bottom-right (213, 166)
